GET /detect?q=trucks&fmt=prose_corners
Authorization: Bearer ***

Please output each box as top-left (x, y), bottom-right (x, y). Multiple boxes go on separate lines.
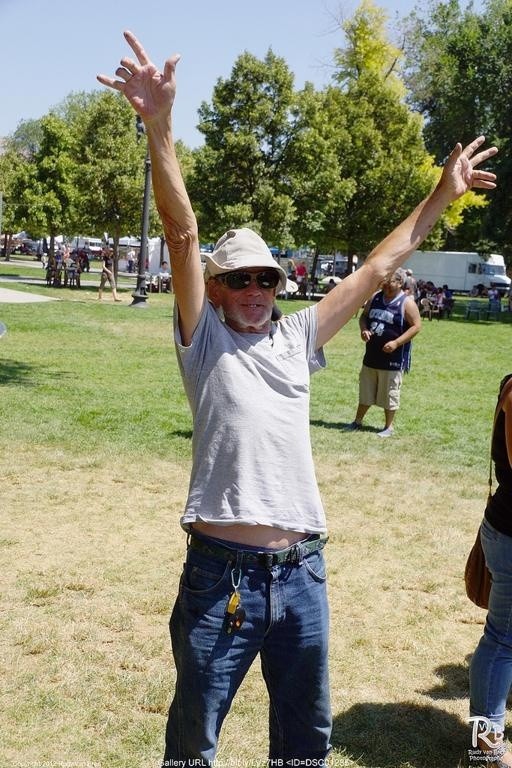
top-left (357, 249), bottom-right (512, 298)
top-left (73, 236), bottom-right (102, 255)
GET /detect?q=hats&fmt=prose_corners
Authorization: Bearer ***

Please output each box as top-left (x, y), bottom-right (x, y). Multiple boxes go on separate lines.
top-left (200, 228), bottom-right (287, 293)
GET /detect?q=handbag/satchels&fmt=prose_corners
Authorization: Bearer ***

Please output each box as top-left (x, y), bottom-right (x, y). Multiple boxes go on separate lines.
top-left (465, 537), bottom-right (490, 609)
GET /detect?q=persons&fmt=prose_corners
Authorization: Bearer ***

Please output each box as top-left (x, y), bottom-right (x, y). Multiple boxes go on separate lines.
top-left (40, 243), bottom-right (171, 301)
top-left (96, 29), bottom-right (499, 767)
top-left (284, 258), bottom-right (500, 324)
top-left (337, 267), bottom-right (422, 438)
top-left (464, 371), bottom-right (512, 768)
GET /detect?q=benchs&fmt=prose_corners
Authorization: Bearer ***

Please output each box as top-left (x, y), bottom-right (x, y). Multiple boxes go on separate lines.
top-left (47, 265), bottom-right (82, 287)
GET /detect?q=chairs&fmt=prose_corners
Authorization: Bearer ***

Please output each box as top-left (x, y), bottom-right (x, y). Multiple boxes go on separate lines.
top-left (145, 272), bottom-right (173, 293)
top-left (466, 300), bottom-right (501, 321)
top-left (419, 296), bottom-right (446, 322)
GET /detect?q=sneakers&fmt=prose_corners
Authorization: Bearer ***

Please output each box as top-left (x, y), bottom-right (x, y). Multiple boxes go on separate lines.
top-left (379, 427), bottom-right (391, 437)
top-left (476, 733), bottom-right (512, 768)
top-left (345, 423), bottom-right (361, 430)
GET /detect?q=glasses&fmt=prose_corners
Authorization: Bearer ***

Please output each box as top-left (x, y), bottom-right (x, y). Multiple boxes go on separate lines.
top-left (216, 271), bottom-right (279, 290)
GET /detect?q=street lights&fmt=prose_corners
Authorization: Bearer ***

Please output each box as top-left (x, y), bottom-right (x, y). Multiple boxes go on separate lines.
top-left (127, 114), bottom-right (151, 310)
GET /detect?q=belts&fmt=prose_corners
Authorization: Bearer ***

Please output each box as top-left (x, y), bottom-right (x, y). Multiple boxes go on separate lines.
top-left (190, 537), bottom-right (328, 567)
